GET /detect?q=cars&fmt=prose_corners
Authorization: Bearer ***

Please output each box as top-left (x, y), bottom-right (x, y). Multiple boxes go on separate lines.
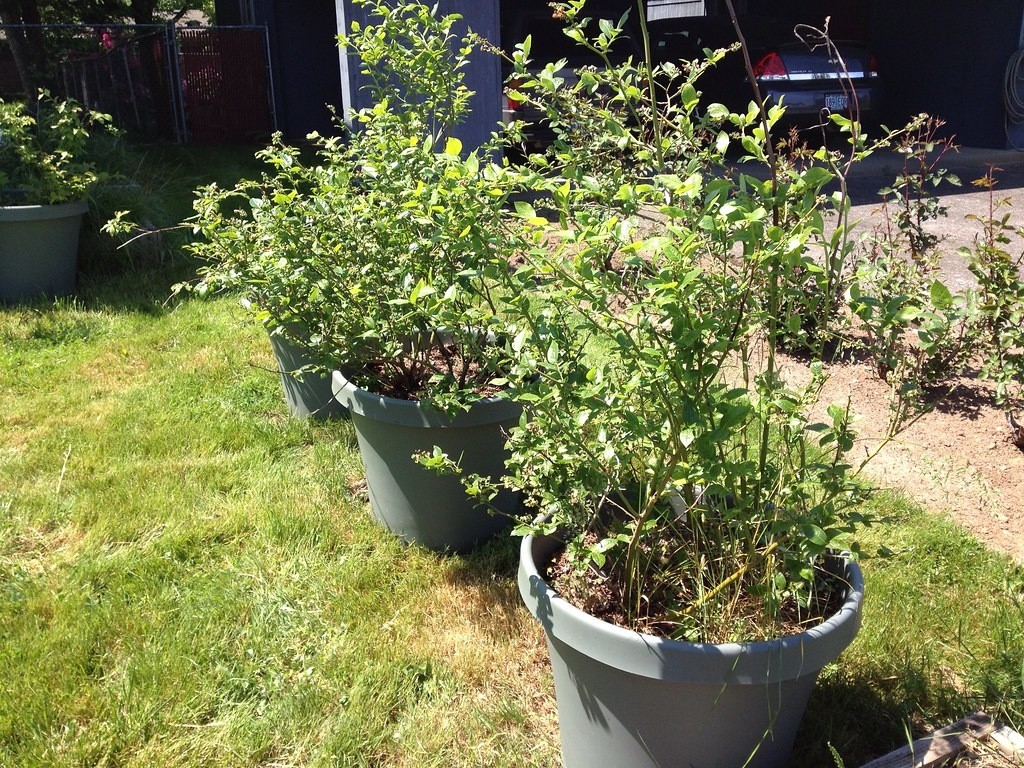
top-left (506, 10), bottom-right (652, 163)
top-left (733, 41), bottom-right (880, 149)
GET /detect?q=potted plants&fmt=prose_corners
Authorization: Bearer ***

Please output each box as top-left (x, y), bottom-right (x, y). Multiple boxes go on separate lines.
top-left (0, 86), bottom-right (119, 304)
top-left (100, 0), bottom-right (984, 768)
